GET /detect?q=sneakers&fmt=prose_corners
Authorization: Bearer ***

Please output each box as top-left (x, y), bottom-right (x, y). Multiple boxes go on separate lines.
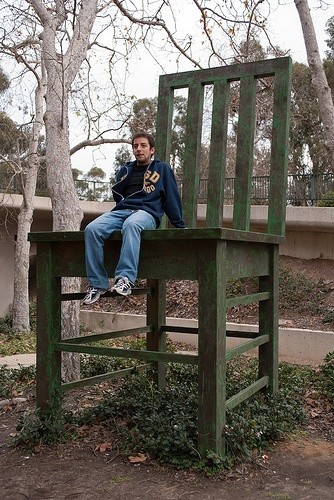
top-left (82, 287), bottom-right (107, 305)
top-left (111, 276), bottom-right (131, 296)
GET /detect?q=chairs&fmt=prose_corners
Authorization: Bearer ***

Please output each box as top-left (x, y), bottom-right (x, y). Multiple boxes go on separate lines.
top-left (27, 57), bottom-right (294, 462)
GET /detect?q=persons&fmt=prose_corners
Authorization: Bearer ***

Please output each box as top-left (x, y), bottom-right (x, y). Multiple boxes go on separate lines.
top-left (82, 131), bottom-right (186, 305)
